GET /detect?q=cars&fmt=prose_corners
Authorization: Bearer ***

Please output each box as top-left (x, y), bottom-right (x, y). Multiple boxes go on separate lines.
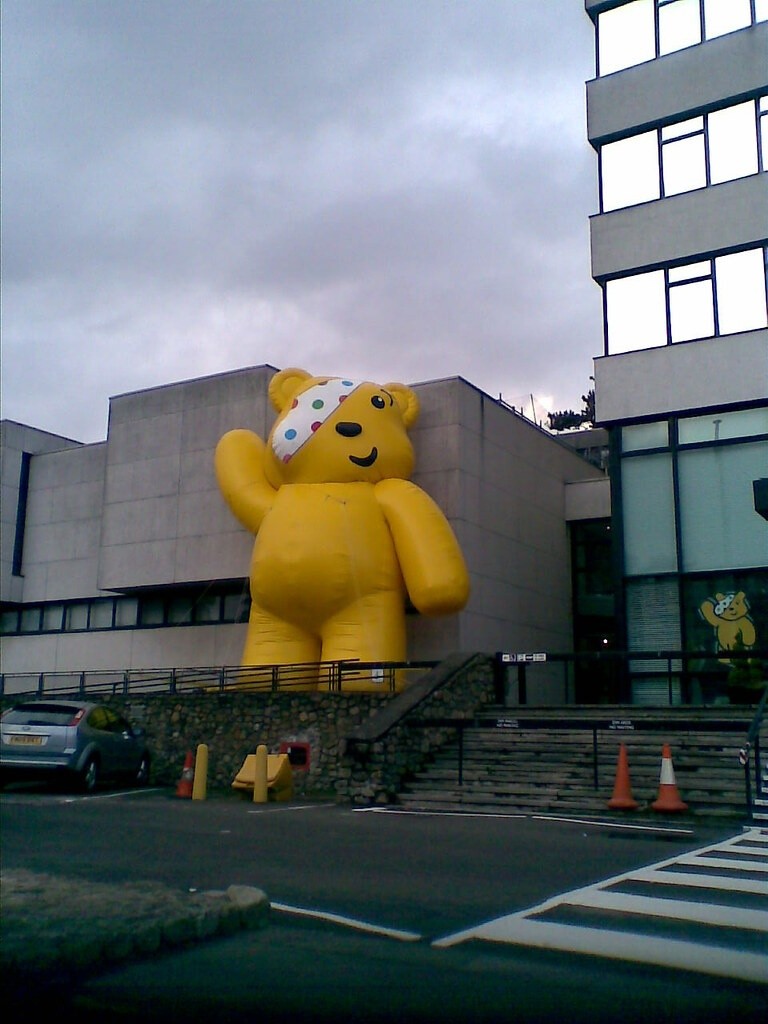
top-left (0, 699), bottom-right (151, 792)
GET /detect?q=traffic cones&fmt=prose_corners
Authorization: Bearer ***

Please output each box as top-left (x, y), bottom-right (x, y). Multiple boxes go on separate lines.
top-left (169, 753), bottom-right (195, 801)
top-left (652, 744), bottom-right (688, 810)
top-left (608, 742), bottom-right (638, 806)
